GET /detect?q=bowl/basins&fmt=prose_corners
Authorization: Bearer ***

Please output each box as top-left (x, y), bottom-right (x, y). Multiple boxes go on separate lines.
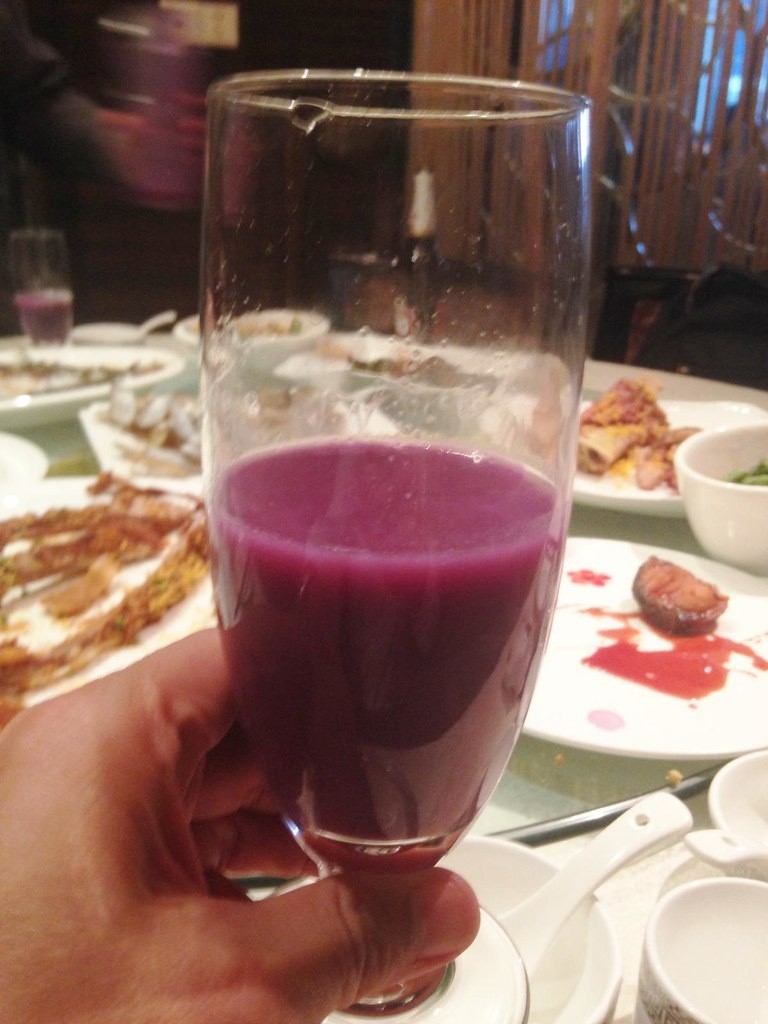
top-left (707, 748), bottom-right (768, 879)
top-left (229, 307), bottom-right (330, 381)
top-left (70, 321), bottom-right (148, 348)
top-left (634, 875), bottom-right (767, 1024)
top-left (673, 419), bottom-right (768, 572)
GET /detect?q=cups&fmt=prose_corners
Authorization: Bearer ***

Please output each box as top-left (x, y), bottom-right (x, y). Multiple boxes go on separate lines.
top-left (7, 227), bottom-right (75, 351)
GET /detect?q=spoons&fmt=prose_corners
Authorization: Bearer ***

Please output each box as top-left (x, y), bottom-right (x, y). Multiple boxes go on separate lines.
top-left (492, 792), bottom-right (694, 974)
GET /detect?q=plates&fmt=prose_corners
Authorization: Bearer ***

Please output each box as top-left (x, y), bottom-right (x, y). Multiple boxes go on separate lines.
top-left (522, 534), bottom-right (768, 760)
top-left (435, 833), bottom-right (623, 1024)
top-left (0, 342), bottom-right (184, 431)
top-left (570, 361), bottom-right (768, 518)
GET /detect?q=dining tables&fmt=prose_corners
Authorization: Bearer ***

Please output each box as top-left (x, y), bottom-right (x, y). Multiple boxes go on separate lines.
top-left (0, 330), bottom-right (768, 1024)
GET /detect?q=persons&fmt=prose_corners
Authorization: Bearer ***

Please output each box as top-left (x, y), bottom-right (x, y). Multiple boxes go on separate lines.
top-left (0, 629), bottom-right (480, 1023)
top-left (0, 0), bottom-right (252, 326)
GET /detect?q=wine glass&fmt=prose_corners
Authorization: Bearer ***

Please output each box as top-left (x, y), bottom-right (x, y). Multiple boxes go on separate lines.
top-left (195, 67), bottom-right (597, 1024)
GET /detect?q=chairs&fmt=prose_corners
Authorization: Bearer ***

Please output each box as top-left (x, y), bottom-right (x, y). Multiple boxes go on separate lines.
top-left (597, 266), bottom-right (768, 397)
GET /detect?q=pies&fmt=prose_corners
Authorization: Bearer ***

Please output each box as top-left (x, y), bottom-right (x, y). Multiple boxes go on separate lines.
top-left (575, 377), bottom-right (654, 473)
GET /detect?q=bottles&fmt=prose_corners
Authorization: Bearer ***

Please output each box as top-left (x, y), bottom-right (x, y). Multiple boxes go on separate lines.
top-left (394, 155), bottom-right (446, 348)
